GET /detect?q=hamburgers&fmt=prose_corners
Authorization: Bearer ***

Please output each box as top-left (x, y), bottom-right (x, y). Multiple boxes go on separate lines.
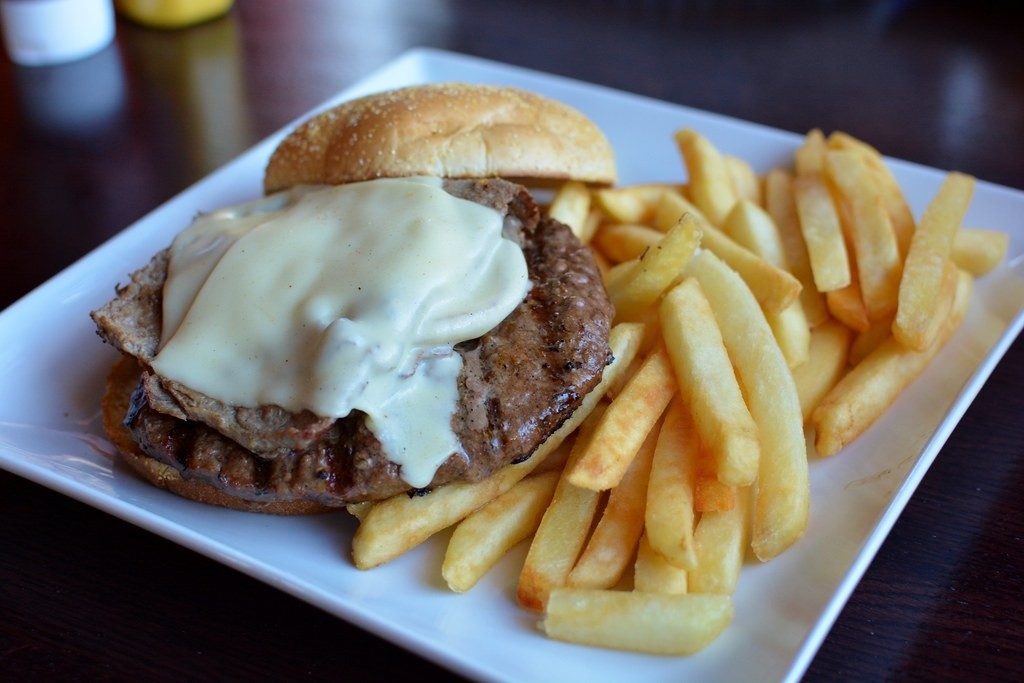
top-left (103, 84), bottom-right (618, 509)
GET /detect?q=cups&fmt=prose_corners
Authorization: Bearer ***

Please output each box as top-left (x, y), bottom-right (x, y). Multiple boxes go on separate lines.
top-left (0, 0), bottom-right (115, 66)
top-left (120, 0), bottom-right (235, 28)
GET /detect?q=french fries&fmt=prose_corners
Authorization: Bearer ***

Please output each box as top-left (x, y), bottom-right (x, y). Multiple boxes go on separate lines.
top-left (353, 127), bottom-right (1010, 651)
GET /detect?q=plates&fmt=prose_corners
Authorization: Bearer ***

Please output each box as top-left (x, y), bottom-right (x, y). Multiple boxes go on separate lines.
top-left (0, 48), bottom-right (1024, 683)
